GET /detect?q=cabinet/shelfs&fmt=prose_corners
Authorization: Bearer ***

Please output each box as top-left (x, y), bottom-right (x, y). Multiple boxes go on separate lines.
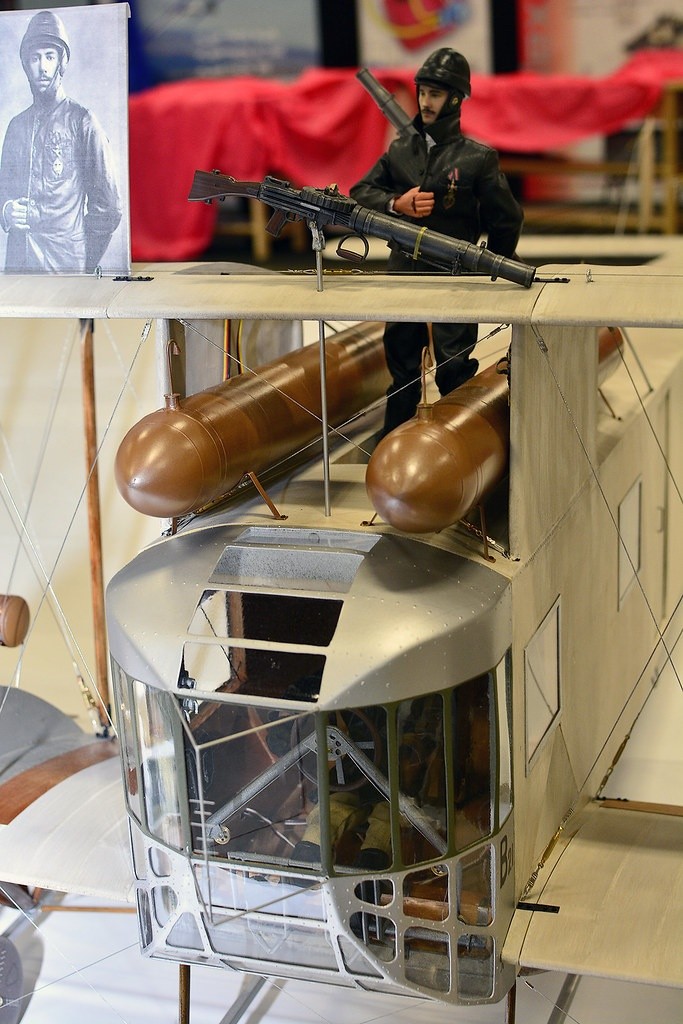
top-left (491, 83), bottom-right (678, 237)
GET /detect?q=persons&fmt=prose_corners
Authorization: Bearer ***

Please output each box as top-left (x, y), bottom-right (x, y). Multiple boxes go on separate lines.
top-left (0, 11), bottom-right (122, 275)
top-left (284, 732), bottom-right (423, 940)
top-left (348, 48), bottom-right (524, 433)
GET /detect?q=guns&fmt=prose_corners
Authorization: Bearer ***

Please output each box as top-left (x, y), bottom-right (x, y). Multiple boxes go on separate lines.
top-left (188, 169), bottom-right (539, 293)
top-left (355, 69), bottom-right (416, 137)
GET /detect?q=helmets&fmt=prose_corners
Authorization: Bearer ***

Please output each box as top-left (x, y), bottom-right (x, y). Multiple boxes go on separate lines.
top-left (20, 11), bottom-right (70, 59)
